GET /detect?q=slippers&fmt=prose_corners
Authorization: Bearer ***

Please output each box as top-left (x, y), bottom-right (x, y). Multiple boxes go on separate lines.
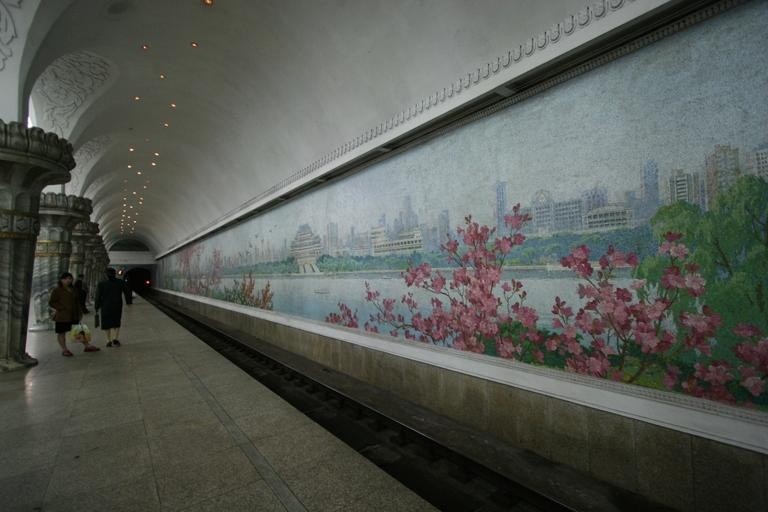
top-left (84, 345), bottom-right (100, 352)
top-left (62, 350), bottom-right (73, 356)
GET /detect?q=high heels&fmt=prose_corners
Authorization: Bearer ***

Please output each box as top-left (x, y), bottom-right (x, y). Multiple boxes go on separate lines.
top-left (106, 339), bottom-right (120, 347)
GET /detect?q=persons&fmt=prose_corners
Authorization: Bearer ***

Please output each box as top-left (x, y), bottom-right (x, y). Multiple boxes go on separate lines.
top-left (94, 268), bottom-right (132, 347)
top-left (123, 276), bottom-right (132, 305)
top-left (48, 273), bottom-right (100, 356)
top-left (72, 273), bottom-right (89, 314)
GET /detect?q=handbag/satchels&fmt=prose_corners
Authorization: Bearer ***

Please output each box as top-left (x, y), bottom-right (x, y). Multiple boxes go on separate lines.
top-left (71, 324), bottom-right (90, 343)
top-left (94, 312), bottom-right (99, 328)
